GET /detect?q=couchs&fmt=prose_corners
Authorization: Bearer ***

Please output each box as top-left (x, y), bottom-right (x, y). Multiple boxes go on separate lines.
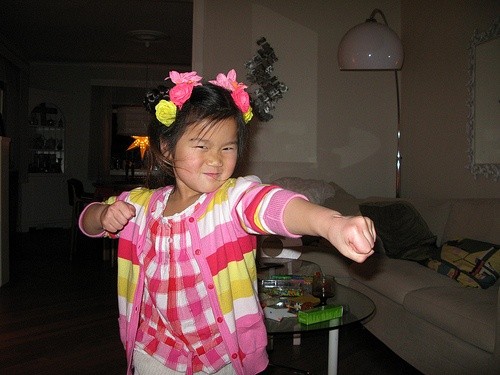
top-left (256, 161), bottom-right (362, 282)
top-left (351, 197), bottom-right (500, 375)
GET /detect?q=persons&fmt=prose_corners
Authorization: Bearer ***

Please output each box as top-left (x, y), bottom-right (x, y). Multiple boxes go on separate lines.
top-left (79, 69), bottom-right (376, 375)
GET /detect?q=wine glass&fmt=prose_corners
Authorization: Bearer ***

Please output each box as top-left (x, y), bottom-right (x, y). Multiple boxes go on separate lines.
top-left (313, 274), bottom-right (336, 307)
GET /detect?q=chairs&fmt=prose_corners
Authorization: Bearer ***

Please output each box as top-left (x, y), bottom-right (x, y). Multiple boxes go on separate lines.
top-left (67, 177), bottom-right (111, 266)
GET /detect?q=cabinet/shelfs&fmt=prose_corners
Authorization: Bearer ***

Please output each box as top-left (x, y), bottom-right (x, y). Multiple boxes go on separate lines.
top-left (26, 101), bottom-right (66, 173)
top-left (18, 172), bottom-right (71, 232)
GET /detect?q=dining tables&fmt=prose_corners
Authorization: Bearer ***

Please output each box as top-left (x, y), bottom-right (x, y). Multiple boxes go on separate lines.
top-left (90, 184), bottom-right (150, 267)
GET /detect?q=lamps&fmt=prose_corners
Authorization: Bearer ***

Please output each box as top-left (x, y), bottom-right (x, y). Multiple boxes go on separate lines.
top-left (337, 8), bottom-right (404, 200)
top-left (116, 40), bottom-right (153, 136)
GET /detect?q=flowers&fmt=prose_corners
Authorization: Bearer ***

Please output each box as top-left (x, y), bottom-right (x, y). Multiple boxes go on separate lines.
top-left (155, 68), bottom-right (254, 127)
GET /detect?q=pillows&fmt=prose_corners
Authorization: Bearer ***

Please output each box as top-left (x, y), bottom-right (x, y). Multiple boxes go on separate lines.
top-left (421, 238), bottom-right (500, 289)
top-left (358, 201), bottom-right (440, 262)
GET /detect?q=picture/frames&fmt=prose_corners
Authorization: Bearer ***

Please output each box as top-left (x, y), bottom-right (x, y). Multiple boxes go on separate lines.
top-left (465, 27), bottom-right (500, 182)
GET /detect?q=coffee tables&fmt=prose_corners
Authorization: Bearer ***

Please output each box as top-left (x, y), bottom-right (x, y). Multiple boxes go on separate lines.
top-left (254, 256), bottom-right (376, 375)
